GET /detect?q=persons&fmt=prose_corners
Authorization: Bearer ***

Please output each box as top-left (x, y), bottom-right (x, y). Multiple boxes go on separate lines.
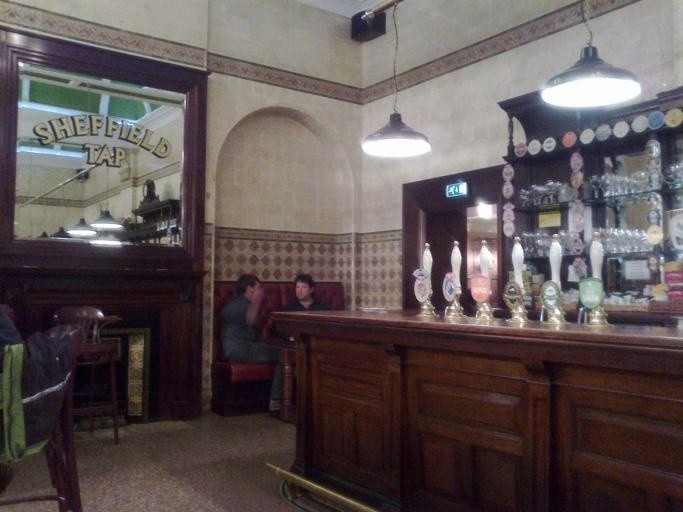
top-left (271, 273), bottom-right (336, 337)
top-left (216, 272), bottom-right (287, 413)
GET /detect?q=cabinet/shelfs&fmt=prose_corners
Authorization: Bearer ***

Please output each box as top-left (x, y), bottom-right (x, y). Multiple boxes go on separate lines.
top-left (498, 85), bottom-right (682, 328)
top-left (128, 197), bottom-right (180, 243)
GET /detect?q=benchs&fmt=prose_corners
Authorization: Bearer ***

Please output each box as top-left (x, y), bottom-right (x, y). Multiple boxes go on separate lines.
top-left (212, 281), bottom-right (347, 416)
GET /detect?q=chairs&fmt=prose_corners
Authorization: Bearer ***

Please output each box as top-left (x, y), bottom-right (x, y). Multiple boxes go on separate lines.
top-left (0, 326), bottom-right (82, 512)
top-left (53, 307), bottom-right (123, 446)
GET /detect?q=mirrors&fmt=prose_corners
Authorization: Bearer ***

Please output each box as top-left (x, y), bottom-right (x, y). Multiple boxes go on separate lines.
top-left (1, 25), bottom-right (213, 260)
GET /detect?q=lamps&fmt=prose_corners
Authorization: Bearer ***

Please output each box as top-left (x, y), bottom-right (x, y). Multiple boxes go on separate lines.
top-left (50, 185), bottom-right (71, 238)
top-left (35, 195), bottom-right (50, 239)
top-left (539, 0), bottom-right (643, 111)
top-left (90, 164), bottom-right (123, 228)
top-left (360, 1), bottom-right (433, 158)
top-left (66, 168), bottom-right (97, 237)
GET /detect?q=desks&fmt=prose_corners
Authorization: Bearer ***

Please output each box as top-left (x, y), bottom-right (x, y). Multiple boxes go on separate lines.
top-left (271, 343), bottom-right (297, 423)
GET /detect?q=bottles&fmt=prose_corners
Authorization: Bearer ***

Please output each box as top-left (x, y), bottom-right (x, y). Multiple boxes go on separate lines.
top-left (664, 162), bottom-right (682, 188)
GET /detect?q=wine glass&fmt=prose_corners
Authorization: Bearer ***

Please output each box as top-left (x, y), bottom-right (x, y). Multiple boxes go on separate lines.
top-left (518, 181), bottom-right (570, 207)
top-left (590, 174), bottom-right (649, 198)
top-left (596, 226), bottom-right (648, 253)
top-left (521, 230), bottom-right (567, 257)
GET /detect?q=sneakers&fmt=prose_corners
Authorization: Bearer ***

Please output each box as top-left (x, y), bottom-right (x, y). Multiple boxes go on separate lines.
top-left (269, 398), bottom-right (284, 411)
